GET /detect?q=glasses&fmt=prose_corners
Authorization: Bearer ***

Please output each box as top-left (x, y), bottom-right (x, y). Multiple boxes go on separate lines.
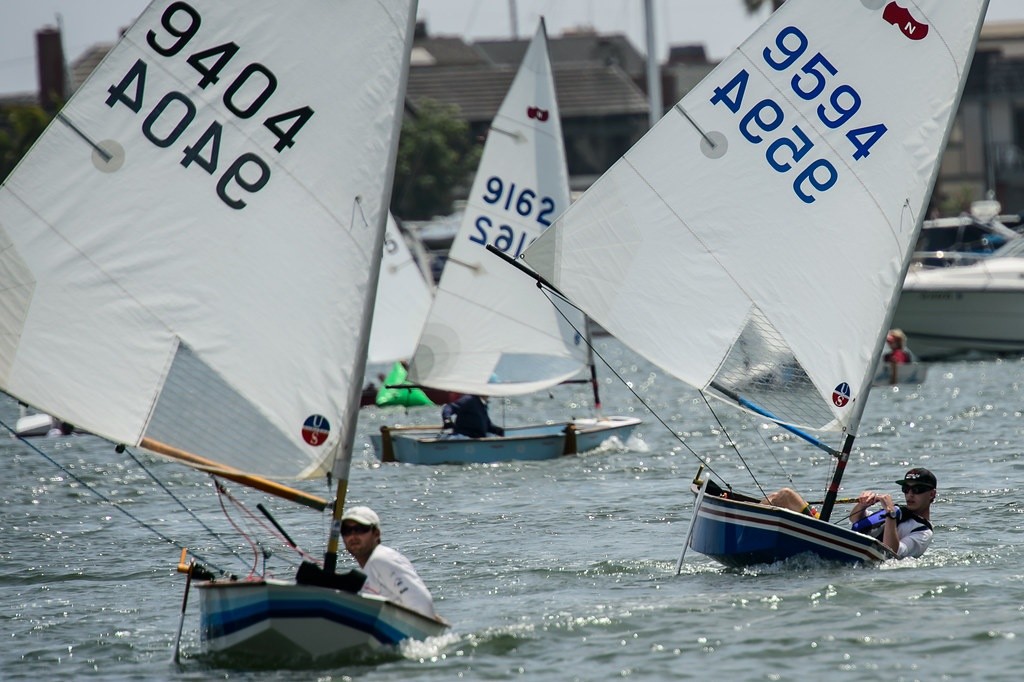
top-left (902, 484), bottom-right (933, 493)
top-left (340, 523), bottom-right (373, 536)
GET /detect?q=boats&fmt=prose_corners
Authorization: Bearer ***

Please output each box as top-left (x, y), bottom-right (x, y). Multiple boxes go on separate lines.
top-left (723, 195), bottom-right (1024, 399)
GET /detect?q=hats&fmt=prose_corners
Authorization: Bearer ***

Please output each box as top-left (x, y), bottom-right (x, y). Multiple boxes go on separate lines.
top-left (886, 335), bottom-right (901, 344)
top-left (896, 468), bottom-right (936, 489)
top-left (342, 506), bottom-right (380, 530)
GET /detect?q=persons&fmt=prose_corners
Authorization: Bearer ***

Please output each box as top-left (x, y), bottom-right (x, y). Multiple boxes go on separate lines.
top-left (882, 330), bottom-right (914, 365)
top-left (761, 468), bottom-right (936, 559)
top-left (341, 506), bottom-right (444, 623)
top-left (442, 374), bottom-right (505, 438)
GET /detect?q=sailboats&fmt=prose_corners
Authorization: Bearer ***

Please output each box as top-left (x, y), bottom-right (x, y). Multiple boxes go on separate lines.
top-left (364, 14), bottom-right (645, 466)
top-left (1, 1), bottom-right (455, 673)
top-left (486, 0), bottom-right (994, 576)
top-left (357, 205), bottom-right (435, 370)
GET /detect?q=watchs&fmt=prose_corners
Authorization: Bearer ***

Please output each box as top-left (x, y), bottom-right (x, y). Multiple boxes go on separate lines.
top-left (884, 509), bottom-right (897, 519)
